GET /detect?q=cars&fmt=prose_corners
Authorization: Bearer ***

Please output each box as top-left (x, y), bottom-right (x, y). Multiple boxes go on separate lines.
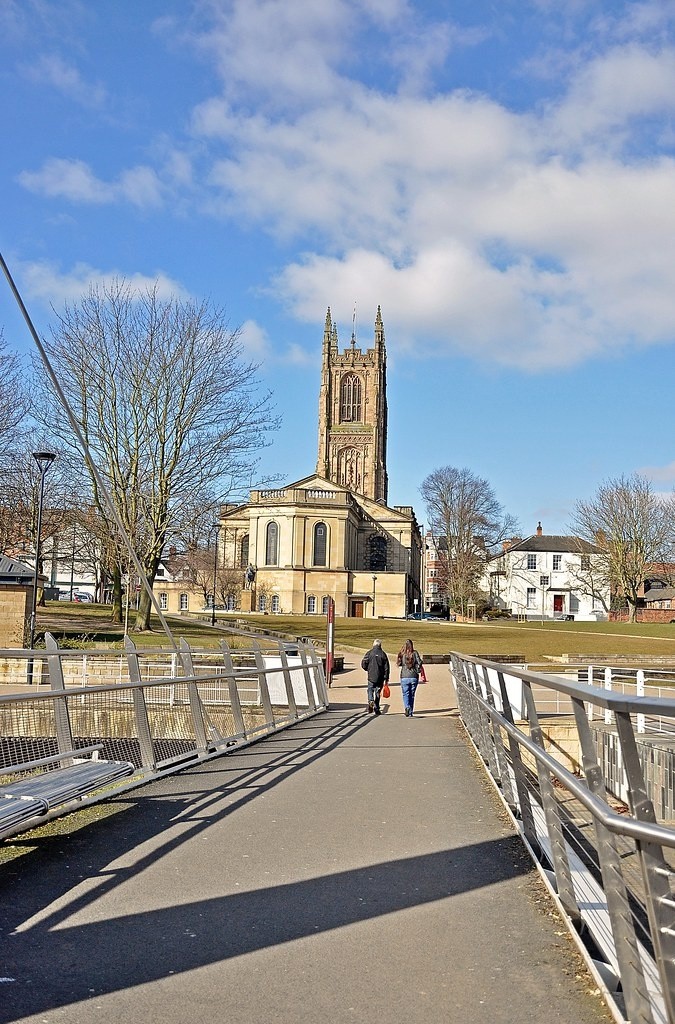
top-left (59, 591), bottom-right (94, 603)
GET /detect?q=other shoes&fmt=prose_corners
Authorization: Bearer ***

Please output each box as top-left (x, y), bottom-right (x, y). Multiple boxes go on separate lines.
top-left (410, 712), bottom-right (413, 717)
top-left (405, 708), bottom-right (410, 717)
top-left (368, 701), bottom-right (374, 713)
top-left (374, 709), bottom-right (381, 715)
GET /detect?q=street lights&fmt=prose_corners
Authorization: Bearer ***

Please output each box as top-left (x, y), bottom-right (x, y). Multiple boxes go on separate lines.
top-left (25, 450), bottom-right (58, 690)
top-left (415, 524), bottom-right (424, 620)
top-left (208, 522), bottom-right (223, 627)
top-left (372, 575), bottom-right (377, 616)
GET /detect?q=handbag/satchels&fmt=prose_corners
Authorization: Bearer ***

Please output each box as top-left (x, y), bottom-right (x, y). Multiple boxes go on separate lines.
top-left (383, 682), bottom-right (390, 698)
top-left (420, 666), bottom-right (426, 683)
top-left (414, 651), bottom-right (423, 672)
